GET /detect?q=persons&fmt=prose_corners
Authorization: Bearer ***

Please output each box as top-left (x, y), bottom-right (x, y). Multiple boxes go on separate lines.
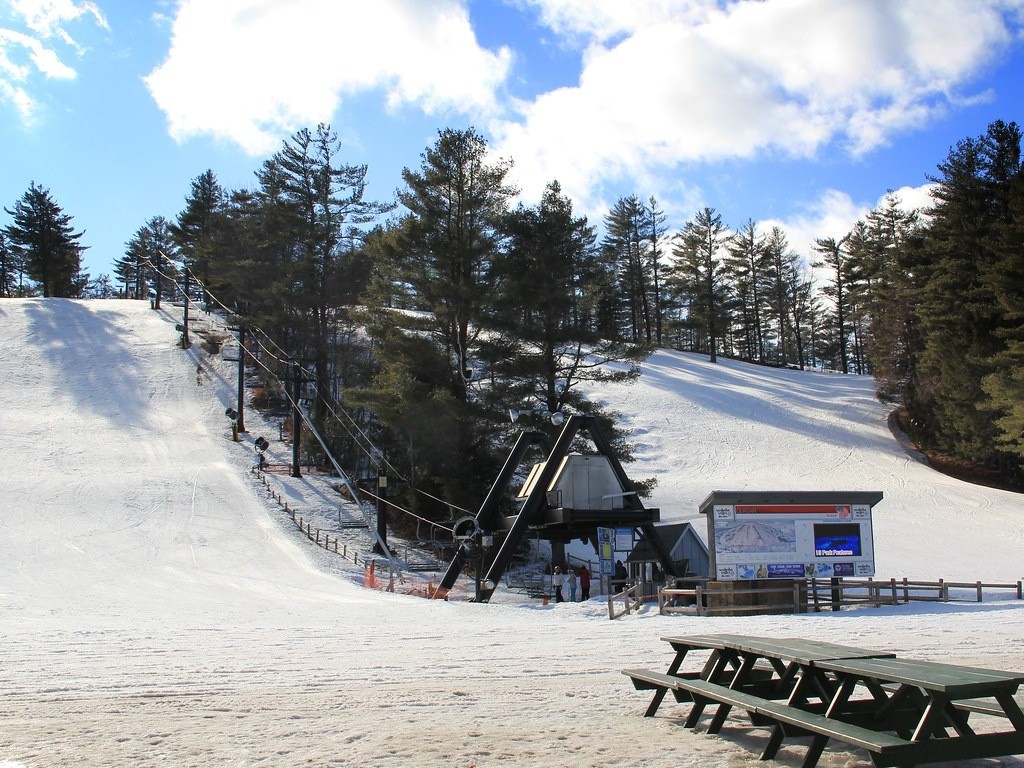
top-left (147, 291), bottom-right (152, 300)
top-left (612, 559), bottom-right (628, 593)
top-left (206, 304), bottom-right (210, 315)
top-left (567, 571), bottom-right (577, 602)
top-left (197, 293), bottom-right (201, 302)
top-left (576, 565), bottom-right (590, 601)
top-left (553, 566), bottom-right (564, 603)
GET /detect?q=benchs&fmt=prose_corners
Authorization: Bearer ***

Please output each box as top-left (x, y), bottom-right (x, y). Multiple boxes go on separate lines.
top-left (622, 632), bottom-right (1024, 768)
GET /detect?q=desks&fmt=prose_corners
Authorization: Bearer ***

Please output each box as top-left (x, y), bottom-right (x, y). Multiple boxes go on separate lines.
top-left (801, 658), bottom-right (1024, 768)
top-left (706, 637), bottom-right (913, 762)
top-left (644, 633), bottom-right (811, 729)
